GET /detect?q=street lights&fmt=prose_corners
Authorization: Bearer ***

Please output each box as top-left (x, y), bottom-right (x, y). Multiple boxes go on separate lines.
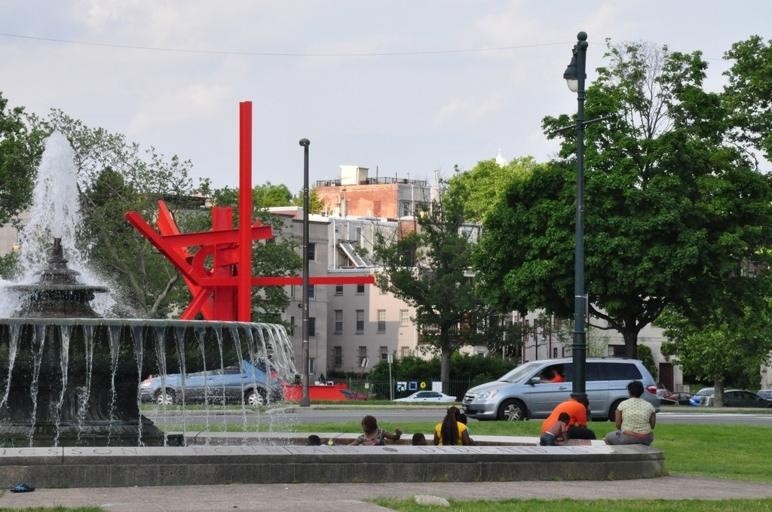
top-left (563, 33), bottom-right (589, 396)
top-left (298, 139), bottom-right (309, 405)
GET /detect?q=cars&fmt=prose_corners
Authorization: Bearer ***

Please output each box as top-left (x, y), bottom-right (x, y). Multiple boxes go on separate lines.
top-left (392, 390), bottom-right (458, 403)
top-left (137, 352), bottom-right (284, 409)
top-left (660, 387), bottom-right (772, 408)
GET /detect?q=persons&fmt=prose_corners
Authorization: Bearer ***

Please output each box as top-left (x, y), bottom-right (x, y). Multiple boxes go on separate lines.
top-left (434, 406), bottom-right (472, 444)
top-left (347, 414), bottom-right (403, 446)
top-left (537, 365), bottom-right (564, 383)
top-left (541, 396), bottom-right (596, 439)
top-left (539, 412), bottom-right (571, 445)
top-left (304, 434), bottom-right (329, 447)
top-left (603, 379), bottom-right (657, 444)
top-left (411, 432), bottom-right (427, 444)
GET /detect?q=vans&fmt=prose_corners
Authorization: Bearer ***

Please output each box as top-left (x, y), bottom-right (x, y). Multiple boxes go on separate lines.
top-left (462, 356), bottom-right (660, 422)
